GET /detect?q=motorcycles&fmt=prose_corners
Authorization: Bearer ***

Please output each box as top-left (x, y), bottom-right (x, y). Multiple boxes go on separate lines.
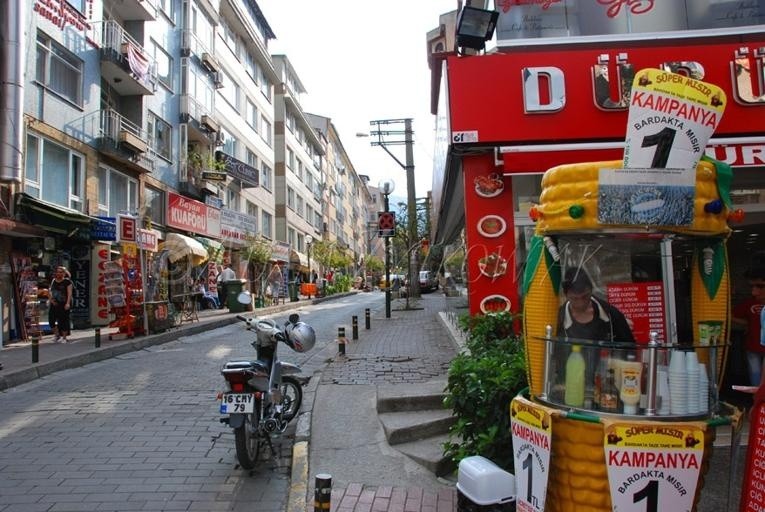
top-left (217, 315), bottom-right (316, 469)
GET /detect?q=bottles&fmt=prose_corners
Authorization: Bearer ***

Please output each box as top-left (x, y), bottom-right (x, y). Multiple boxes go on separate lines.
top-left (563, 345), bottom-right (586, 407)
top-left (593, 347), bottom-right (644, 415)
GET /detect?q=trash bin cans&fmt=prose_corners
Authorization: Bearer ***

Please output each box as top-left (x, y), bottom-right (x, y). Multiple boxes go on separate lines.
top-left (288, 281), bottom-right (299, 301)
top-left (224, 279), bottom-right (247, 313)
top-left (456, 455), bottom-right (516, 512)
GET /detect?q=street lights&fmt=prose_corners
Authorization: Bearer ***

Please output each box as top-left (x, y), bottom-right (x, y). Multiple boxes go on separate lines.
top-left (304, 235), bottom-right (312, 298)
top-left (378, 177), bottom-right (395, 318)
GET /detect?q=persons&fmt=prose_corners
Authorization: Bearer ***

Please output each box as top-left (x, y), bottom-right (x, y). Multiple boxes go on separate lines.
top-left (51, 266), bottom-right (72, 343)
top-left (730, 296), bottom-right (765, 386)
top-left (266, 282), bottom-right (275, 303)
top-left (190, 264), bottom-right (236, 309)
top-left (555, 266), bottom-right (639, 386)
top-left (48, 264), bottom-right (71, 340)
top-left (744, 264), bottom-right (765, 387)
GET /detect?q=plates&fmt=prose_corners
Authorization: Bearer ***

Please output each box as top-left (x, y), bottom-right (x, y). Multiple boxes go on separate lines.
top-left (479, 294), bottom-right (513, 318)
top-left (478, 254), bottom-right (508, 279)
top-left (474, 172), bottom-right (505, 198)
top-left (476, 214), bottom-right (507, 239)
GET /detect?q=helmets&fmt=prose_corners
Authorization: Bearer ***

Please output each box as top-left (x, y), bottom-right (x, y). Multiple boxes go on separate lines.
top-left (284, 321), bottom-right (316, 353)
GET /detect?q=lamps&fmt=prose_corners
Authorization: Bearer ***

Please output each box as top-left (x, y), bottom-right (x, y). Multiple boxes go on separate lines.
top-left (453, 6), bottom-right (500, 57)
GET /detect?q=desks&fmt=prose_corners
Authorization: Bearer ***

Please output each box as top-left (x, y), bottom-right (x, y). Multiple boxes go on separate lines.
top-left (173, 292), bottom-right (204, 327)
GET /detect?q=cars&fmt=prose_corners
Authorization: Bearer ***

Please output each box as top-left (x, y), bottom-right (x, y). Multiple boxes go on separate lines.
top-left (379, 270), bottom-right (439, 293)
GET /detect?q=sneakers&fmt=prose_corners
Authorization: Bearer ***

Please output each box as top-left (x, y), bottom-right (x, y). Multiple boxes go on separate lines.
top-left (49, 334), bottom-right (67, 344)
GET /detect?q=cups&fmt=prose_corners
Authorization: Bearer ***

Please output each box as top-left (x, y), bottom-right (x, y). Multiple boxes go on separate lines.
top-left (655, 350), bottom-right (710, 416)
